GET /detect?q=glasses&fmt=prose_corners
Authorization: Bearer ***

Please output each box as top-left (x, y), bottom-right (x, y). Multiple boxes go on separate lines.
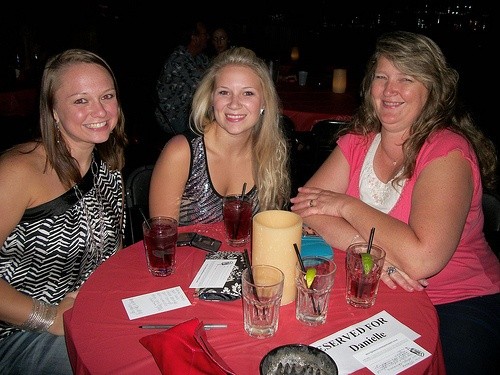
top-left (191, 30), bottom-right (210, 39)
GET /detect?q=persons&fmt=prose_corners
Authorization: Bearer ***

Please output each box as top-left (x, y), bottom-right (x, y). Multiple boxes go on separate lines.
top-left (207, 25), bottom-right (235, 59)
top-left (147, 44), bottom-right (292, 229)
top-left (287, 28), bottom-right (500, 375)
top-left (0, 46), bottom-right (130, 375)
top-left (154, 15), bottom-right (213, 135)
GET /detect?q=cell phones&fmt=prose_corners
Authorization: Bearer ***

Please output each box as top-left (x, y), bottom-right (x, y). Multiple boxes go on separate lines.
top-left (175, 232), bottom-right (222, 253)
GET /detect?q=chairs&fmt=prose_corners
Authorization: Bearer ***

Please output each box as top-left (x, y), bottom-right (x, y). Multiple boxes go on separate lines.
top-left (123, 114), bottom-right (352, 250)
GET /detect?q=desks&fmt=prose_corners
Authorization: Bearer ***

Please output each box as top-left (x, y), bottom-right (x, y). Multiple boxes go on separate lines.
top-left (62, 223), bottom-right (446, 375)
top-left (1, 72), bottom-right (39, 118)
top-left (279, 80), bottom-right (366, 132)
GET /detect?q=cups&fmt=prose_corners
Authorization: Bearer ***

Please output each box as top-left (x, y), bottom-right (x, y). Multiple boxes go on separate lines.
top-left (299, 71), bottom-right (307, 85)
top-left (346, 243), bottom-right (386, 309)
top-left (242, 266), bottom-right (285, 339)
top-left (143, 216), bottom-right (178, 278)
top-left (223, 194), bottom-right (253, 248)
top-left (295, 256), bottom-right (337, 325)
top-left (252, 209), bottom-right (302, 306)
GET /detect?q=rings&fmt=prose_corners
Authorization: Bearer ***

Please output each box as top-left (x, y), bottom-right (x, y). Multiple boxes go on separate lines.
top-left (309, 199), bottom-right (315, 208)
top-left (384, 264), bottom-right (397, 275)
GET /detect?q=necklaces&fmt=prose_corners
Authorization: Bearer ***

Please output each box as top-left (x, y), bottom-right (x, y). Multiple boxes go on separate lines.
top-left (70, 149), bottom-right (107, 296)
top-left (388, 155), bottom-right (404, 168)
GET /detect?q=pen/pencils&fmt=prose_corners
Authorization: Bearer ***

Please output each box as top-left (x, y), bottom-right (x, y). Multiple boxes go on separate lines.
top-left (139, 325), bottom-right (228, 329)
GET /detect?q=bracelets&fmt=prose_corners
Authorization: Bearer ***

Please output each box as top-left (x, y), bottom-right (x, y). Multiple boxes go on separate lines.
top-left (19, 296), bottom-right (58, 336)
top-left (349, 231), bottom-right (362, 247)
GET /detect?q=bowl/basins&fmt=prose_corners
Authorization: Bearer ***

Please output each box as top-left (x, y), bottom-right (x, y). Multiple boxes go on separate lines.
top-left (259, 343), bottom-right (338, 375)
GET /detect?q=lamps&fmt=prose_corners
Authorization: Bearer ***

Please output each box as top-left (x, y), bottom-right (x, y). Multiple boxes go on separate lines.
top-left (291, 47), bottom-right (299, 60)
top-left (333, 68), bottom-right (346, 92)
